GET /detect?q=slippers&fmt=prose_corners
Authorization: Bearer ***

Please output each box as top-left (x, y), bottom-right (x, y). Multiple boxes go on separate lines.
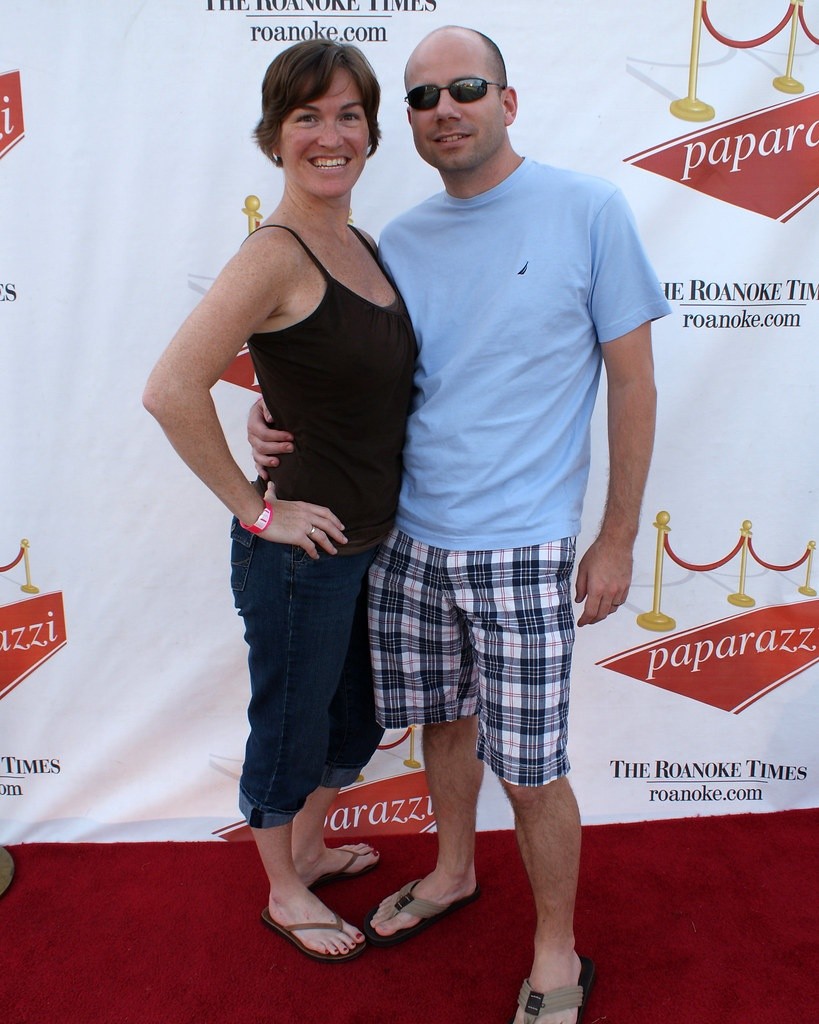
top-left (510, 955), bottom-right (596, 1024)
top-left (300, 843), bottom-right (381, 891)
top-left (363, 878), bottom-right (481, 945)
top-left (260, 902), bottom-right (366, 962)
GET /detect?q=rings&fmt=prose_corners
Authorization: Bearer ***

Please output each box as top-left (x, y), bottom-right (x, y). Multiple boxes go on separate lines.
top-left (307, 525), bottom-right (315, 538)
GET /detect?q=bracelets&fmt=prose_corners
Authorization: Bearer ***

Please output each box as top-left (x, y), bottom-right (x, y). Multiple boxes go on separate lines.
top-left (240, 499), bottom-right (273, 534)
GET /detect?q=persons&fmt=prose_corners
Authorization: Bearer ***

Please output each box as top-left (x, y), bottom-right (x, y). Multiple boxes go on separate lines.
top-left (142, 39), bottom-right (418, 962)
top-left (247, 25), bottom-right (671, 1024)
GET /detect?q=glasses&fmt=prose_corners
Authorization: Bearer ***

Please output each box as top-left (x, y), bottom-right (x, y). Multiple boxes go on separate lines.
top-left (404, 78), bottom-right (506, 111)
